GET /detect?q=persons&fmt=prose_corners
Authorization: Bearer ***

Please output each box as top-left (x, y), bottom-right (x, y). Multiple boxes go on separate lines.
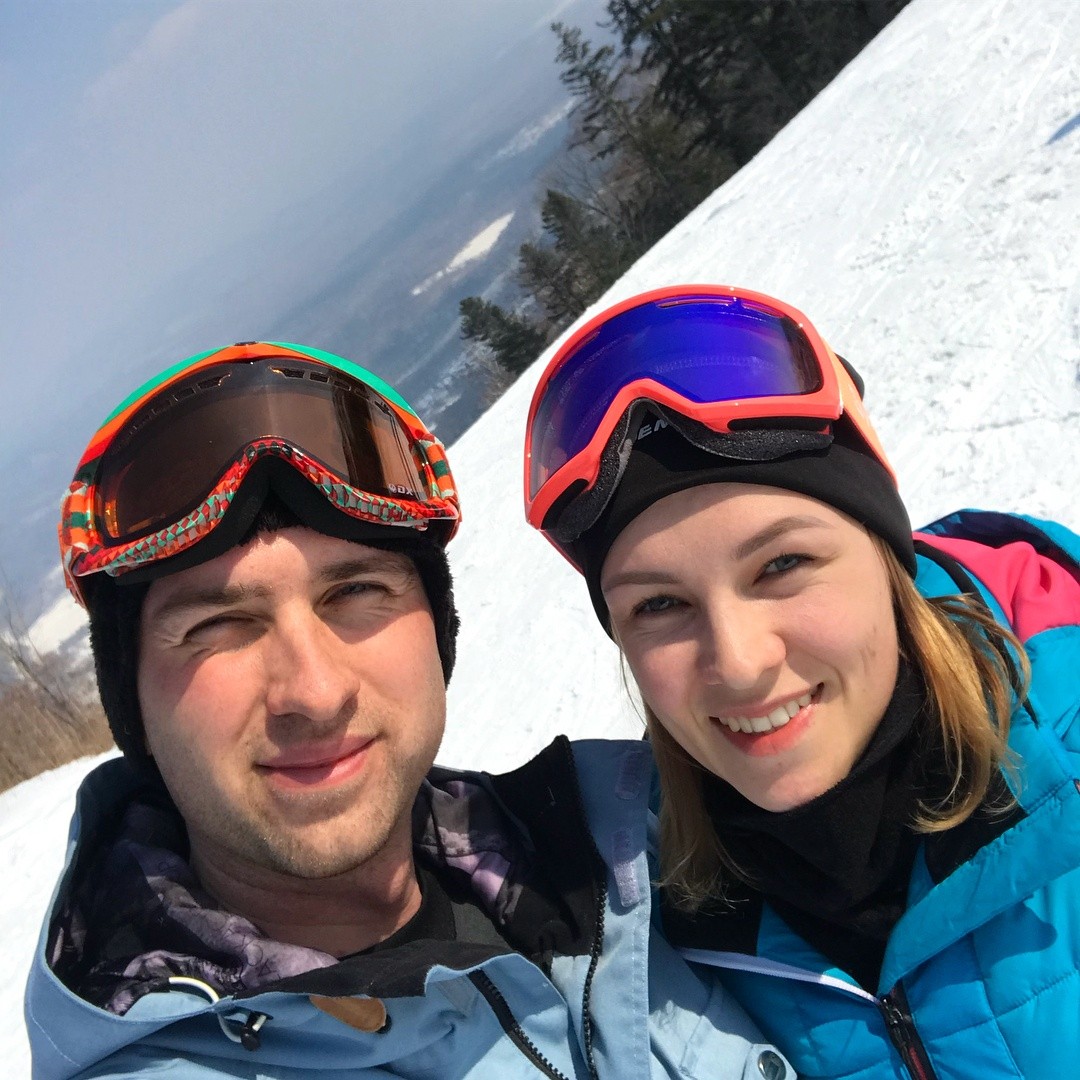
top-left (23, 340), bottom-right (797, 1080)
top-left (523, 285), bottom-right (1080, 1080)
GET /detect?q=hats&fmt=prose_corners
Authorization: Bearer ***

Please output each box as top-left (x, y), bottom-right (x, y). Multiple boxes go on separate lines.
top-left (572, 354), bottom-right (920, 639)
top-left (80, 353), bottom-right (461, 784)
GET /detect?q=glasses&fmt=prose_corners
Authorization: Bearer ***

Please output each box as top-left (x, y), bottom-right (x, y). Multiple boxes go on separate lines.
top-left (512, 287), bottom-right (902, 566)
top-left (56, 342), bottom-right (465, 604)
